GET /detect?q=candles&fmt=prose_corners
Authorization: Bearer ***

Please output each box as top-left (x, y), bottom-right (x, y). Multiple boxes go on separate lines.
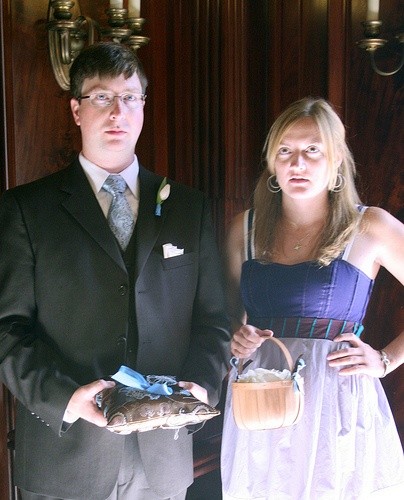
top-left (110, 0), bottom-right (123, 9)
top-left (366, 0), bottom-right (379, 21)
top-left (128, 0), bottom-right (141, 17)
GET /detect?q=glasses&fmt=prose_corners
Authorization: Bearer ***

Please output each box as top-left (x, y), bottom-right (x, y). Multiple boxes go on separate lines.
top-left (79, 93), bottom-right (146, 108)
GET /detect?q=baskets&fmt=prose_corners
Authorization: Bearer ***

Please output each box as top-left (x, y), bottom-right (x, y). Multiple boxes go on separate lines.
top-left (231, 334), bottom-right (304, 431)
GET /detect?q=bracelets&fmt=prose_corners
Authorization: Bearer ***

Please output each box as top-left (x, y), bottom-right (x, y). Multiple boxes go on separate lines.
top-left (377, 348), bottom-right (390, 379)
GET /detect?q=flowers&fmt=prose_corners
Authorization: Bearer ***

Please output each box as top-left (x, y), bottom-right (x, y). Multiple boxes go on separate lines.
top-left (155, 177), bottom-right (170, 216)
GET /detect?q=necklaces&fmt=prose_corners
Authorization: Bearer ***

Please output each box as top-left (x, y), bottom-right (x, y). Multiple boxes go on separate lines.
top-left (287, 226), bottom-right (313, 250)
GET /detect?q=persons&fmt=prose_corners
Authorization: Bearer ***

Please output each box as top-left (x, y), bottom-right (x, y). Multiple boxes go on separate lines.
top-left (217, 97), bottom-right (404, 500)
top-left (0, 39), bottom-right (233, 500)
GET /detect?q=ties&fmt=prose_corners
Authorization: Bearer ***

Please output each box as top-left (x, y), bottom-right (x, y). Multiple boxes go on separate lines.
top-left (102, 174), bottom-right (134, 254)
